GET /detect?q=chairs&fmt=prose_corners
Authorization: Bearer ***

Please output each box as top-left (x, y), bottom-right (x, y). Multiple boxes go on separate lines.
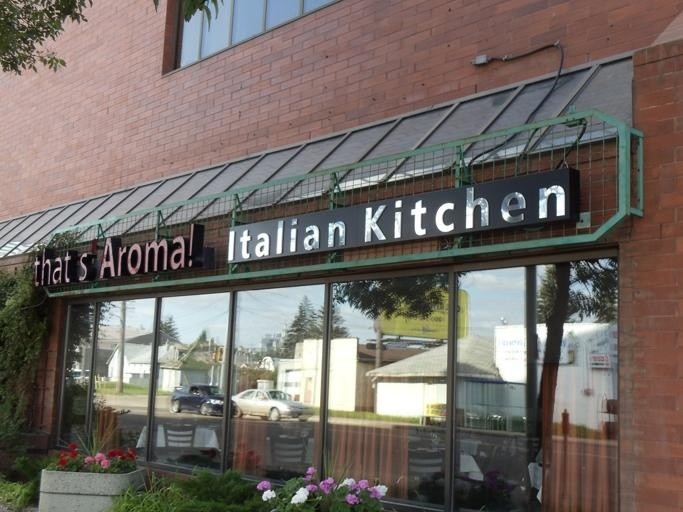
top-left (163, 422), bottom-right (216, 464)
top-left (266, 433), bottom-right (310, 477)
top-left (408, 450), bottom-right (444, 487)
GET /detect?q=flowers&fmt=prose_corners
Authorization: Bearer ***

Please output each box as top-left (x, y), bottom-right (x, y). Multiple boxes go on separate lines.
top-left (46, 444), bottom-right (137, 473)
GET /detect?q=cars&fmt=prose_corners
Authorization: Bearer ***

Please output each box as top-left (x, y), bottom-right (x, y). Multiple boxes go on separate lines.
top-left (69, 369), bottom-right (90, 384)
top-left (231, 388), bottom-right (305, 421)
top-left (170, 386), bottom-right (236, 418)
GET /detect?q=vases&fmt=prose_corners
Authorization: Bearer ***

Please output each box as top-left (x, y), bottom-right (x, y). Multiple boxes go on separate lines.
top-left (38, 468), bottom-right (147, 512)
